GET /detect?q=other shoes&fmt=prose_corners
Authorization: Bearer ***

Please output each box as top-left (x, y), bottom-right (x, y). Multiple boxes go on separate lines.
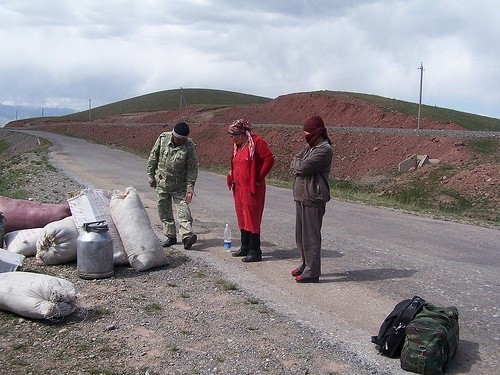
top-left (184, 235), bottom-right (197, 249)
top-left (163, 238), bottom-right (177, 247)
top-left (295, 276), bottom-right (319, 283)
top-left (291, 268), bottom-right (302, 277)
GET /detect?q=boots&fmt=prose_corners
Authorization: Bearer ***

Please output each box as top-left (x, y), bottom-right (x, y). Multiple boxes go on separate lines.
top-left (232, 229), bottom-right (247, 257)
top-left (242, 232), bottom-right (262, 262)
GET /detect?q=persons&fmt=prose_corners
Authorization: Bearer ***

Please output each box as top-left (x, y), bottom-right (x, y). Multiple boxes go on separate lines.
top-left (226, 118), bottom-right (274, 262)
top-left (147, 122), bottom-right (200, 250)
top-left (289, 115), bottom-right (333, 283)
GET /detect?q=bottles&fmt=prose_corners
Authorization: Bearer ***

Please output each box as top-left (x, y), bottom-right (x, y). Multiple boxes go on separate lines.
top-left (224, 224), bottom-right (232, 252)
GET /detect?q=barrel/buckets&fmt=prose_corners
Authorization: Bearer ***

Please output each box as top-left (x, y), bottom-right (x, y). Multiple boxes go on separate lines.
top-left (76, 220), bottom-right (114, 280)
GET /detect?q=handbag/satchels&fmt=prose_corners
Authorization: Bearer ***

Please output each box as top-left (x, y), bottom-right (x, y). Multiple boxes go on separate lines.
top-left (400, 304), bottom-right (460, 375)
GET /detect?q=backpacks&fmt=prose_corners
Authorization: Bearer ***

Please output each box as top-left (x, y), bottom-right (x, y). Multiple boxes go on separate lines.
top-left (371, 295), bottom-right (426, 358)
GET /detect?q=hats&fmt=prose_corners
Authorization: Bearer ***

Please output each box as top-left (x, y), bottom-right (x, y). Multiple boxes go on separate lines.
top-left (173, 122), bottom-right (189, 140)
